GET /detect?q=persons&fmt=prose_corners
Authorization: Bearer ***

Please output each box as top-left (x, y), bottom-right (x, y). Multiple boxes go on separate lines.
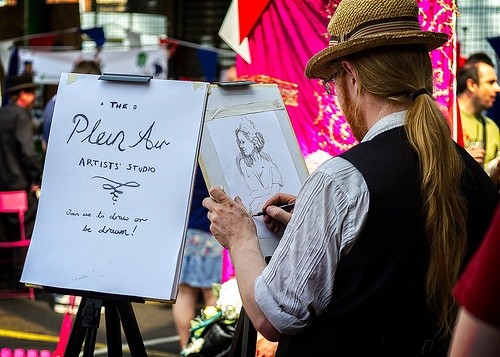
top-left (40, 60), bottom-right (102, 150)
top-left (448, 208), bottom-right (500, 357)
top-left (0, 76), bottom-right (55, 308)
top-left (457, 53), bottom-right (500, 177)
top-left (172, 163), bottom-right (224, 350)
top-left (203, 0), bottom-right (498, 357)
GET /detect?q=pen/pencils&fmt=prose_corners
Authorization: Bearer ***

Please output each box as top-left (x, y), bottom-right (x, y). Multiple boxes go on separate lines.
top-left (253, 202), bottom-right (295, 216)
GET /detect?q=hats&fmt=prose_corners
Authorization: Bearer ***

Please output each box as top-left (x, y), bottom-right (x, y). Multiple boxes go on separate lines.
top-left (4, 76), bottom-right (40, 95)
top-left (305, 0), bottom-right (449, 79)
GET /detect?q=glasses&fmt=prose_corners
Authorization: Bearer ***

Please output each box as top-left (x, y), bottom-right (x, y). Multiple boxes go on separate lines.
top-left (322, 70), bottom-right (341, 96)
